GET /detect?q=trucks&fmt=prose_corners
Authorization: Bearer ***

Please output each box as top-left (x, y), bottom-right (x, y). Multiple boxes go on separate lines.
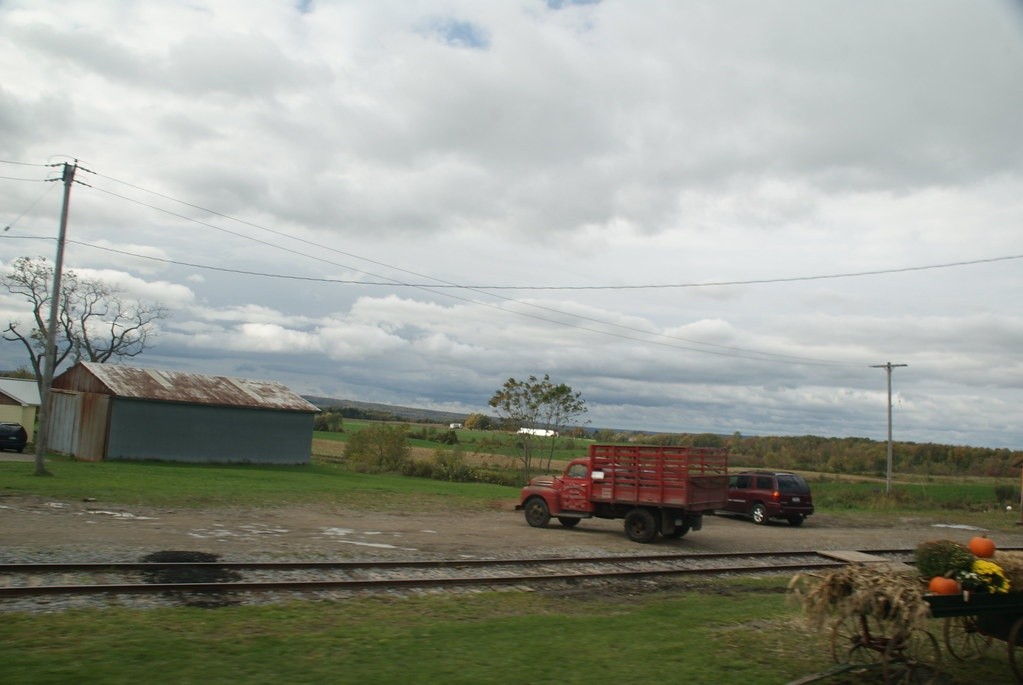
top-left (515, 444), bottom-right (730, 543)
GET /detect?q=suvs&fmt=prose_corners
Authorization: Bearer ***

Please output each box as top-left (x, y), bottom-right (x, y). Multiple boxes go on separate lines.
top-left (704, 471), bottom-right (814, 526)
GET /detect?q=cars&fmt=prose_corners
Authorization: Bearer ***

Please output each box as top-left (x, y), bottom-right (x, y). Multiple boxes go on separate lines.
top-left (0, 421), bottom-right (27, 453)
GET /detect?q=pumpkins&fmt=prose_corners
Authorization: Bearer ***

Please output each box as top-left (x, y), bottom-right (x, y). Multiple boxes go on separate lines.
top-left (928, 576), bottom-right (958, 595)
top-left (969, 533), bottom-right (995, 558)
